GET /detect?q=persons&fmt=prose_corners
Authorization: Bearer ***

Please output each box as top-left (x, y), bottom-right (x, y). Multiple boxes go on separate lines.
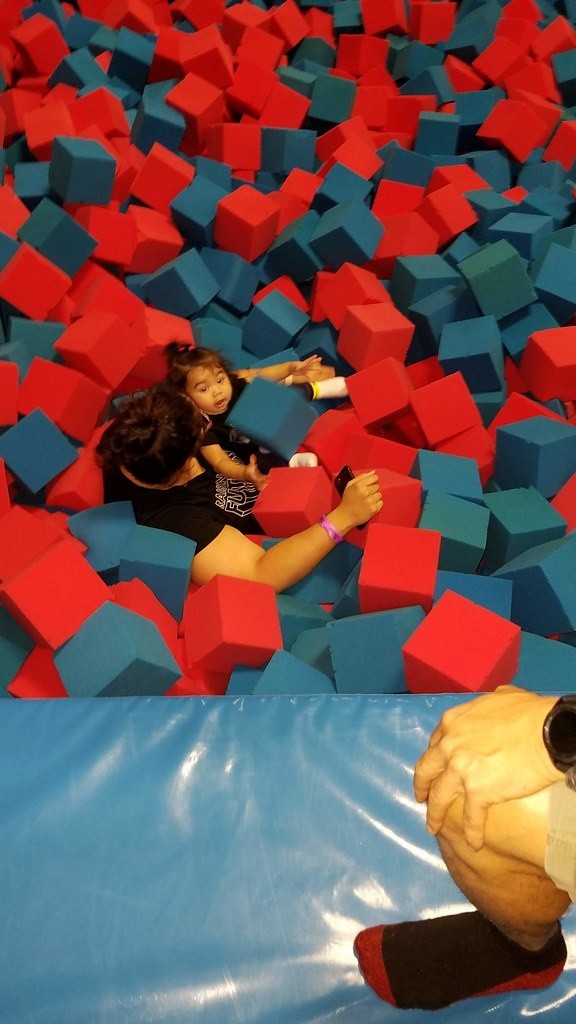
top-left (99, 361), bottom-right (383, 594)
top-left (165, 341), bottom-right (322, 491)
top-left (354, 684), bottom-right (576, 1011)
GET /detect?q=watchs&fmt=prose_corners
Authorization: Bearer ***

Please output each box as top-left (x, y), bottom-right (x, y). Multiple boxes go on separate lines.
top-left (542, 694), bottom-right (576, 774)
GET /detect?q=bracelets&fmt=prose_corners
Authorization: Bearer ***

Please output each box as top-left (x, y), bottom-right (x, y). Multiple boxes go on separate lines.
top-left (320, 517), bottom-right (343, 544)
top-left (285, 375), bottom-right (292, 387)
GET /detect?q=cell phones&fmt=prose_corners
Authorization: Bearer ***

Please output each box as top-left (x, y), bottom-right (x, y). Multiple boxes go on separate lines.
top-left (334, 466), bottom-right (372, 530)
